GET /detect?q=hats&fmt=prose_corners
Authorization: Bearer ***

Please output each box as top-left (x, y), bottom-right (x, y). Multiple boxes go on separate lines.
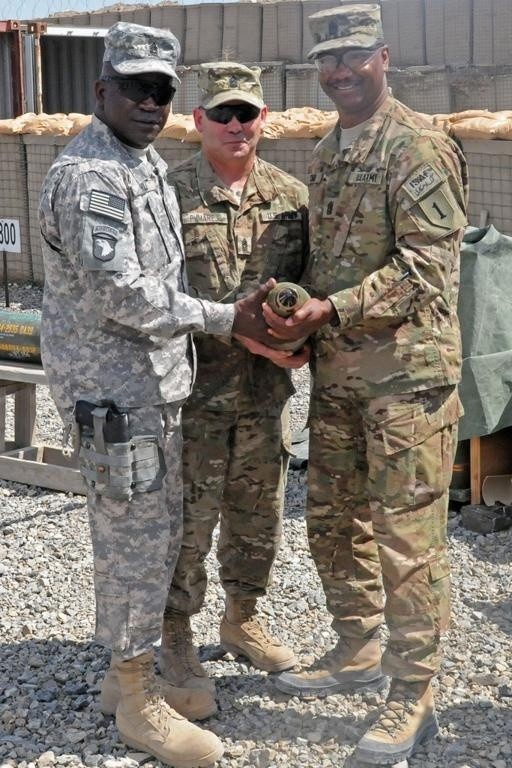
top-left (306, 4), bottom-right (383, 60)
top-left (198, 63), bottom-right (265, 110)
top-left (102, 22), bottom-right (182, 85)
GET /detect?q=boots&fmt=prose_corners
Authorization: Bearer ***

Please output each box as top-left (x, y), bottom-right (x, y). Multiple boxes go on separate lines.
top-left (100, 648), bottom-right (220, 719)
top-left (278, 630), bottom-right (388, 696)
top-left (113, 654), bottom-right (227, 764)
top-left (156, 616), bottom-right (217, 694)
top-left (356, 680), bottom-right (439, 765)
top-left (222, 595), bottom-right (297, 673)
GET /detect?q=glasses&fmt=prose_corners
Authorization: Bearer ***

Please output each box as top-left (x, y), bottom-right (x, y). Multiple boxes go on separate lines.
top-left (100, 75), bottom-right (176, 105)
top-left (204, 103), bottom-right (260, 124)
top-left (314, 49), bottom-right (380, 73)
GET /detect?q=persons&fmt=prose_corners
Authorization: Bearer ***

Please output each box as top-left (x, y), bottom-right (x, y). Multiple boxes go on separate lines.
top-left (155, 59), bottom-right (311, 699)
top-left (260, 2), bottom-right (469, 765)
top-left (36, 20), bottom-right (305, 765)
